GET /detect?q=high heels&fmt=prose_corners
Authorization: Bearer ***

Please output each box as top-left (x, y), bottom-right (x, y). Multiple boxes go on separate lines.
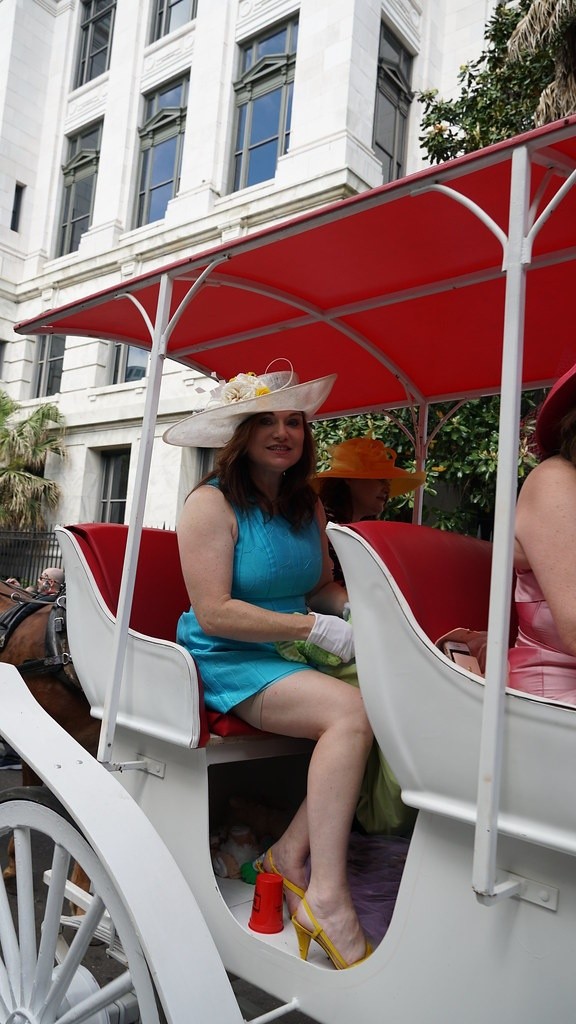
top-left (292, 898), bottom-right (373, 970)
top-left (254, 848), bottom-right (306, 915)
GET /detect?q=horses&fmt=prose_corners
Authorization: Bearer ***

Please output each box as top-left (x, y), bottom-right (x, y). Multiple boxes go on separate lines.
top-left (0, 582), bottom-right (106, 946)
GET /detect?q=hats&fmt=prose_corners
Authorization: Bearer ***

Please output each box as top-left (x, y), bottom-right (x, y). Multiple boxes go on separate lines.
top-left (163, 358), bottom-right (337, 448)
top-left (535, 363), bottom-right (576, 455)
top-left (307, 439), bottom-right (426, 496)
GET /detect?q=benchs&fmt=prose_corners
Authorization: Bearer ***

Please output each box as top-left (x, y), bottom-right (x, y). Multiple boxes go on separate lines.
top-left (49, 511), bottom-right (317, 767)
top-left (323, 509), bottom-right (576, 859)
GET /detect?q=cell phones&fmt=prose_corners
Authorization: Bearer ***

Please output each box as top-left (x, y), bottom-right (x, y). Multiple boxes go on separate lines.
top-left (444, 642), bottom-right (471, 663)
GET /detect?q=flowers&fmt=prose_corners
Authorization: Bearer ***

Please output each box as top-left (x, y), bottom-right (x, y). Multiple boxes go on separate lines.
top-left (172, 367), bottom-right (276, 427)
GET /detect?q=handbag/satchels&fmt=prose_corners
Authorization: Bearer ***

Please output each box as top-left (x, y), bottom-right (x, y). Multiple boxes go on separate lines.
top-left (276, 612), bottom-right (402, 833)
top-left (435, 627), bottom-right (487, 678)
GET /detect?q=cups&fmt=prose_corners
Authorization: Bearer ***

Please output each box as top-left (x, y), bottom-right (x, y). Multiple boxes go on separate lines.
top-left (249, 874), bottom-right (284, 935)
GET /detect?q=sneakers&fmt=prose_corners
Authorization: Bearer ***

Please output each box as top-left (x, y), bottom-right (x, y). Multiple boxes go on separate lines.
top-left (0, 751), bottom-right (22, 770)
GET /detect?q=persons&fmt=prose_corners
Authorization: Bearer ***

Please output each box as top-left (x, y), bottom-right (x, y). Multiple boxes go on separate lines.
top-left (26, 585), bottom-right (38, 593)
top-left (508, 358), bottom-right (576, 704)
top-left (5, 567), bottom-right (65, 596)
top-left (313, 438), bottom-right (426, 849)
top-left (177, 411), bottom-right (372, 971)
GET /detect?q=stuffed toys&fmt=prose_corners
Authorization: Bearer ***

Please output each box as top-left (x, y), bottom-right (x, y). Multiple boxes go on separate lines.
top-left (210, 792), bottom-right (297, 882)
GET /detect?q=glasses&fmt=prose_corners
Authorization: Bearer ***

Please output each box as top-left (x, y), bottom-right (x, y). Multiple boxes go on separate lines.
top-left (40, 577), bottom-right (56, 583)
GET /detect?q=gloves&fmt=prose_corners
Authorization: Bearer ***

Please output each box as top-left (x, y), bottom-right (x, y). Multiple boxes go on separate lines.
top-left (307, 611), bottom-right (355, 664)
